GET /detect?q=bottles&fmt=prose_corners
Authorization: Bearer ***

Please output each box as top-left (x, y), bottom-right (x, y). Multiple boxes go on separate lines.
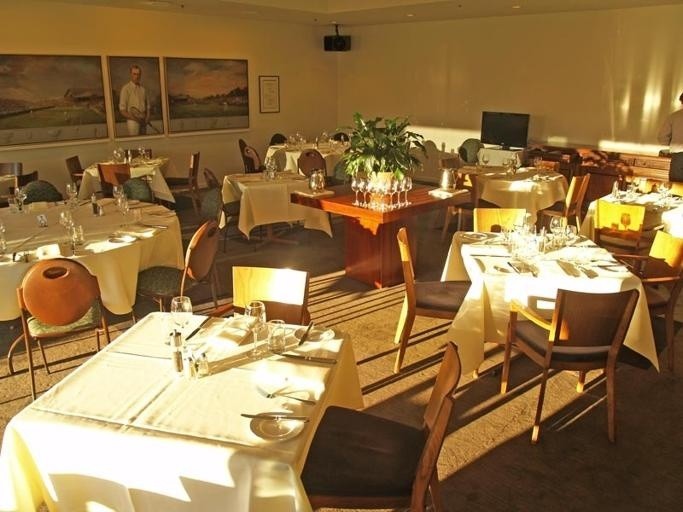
top-left (169, 331), bottom-right (211, 377)
top-left (91, 193), bottom-right (105, 216)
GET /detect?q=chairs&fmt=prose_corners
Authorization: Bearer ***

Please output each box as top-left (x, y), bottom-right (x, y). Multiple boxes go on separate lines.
top-left (204, 132), bottom-right (349, 253)
top-left (593, 175), bottom-right (683, 286)
top-left (431, 156), bottom-right (592, 242)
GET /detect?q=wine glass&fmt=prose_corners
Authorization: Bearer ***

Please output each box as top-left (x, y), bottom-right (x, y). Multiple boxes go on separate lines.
top-left (1, 217), bottom-right (8, 256)
top-left (260, 156), bottom-right (282, 183)
top-left (171, 295), bottom-right (194, 337)
top-left (7, 187), bottom-right (29, 212)
top-left (348, 176), bottom-right (413, 210)
top-left (112, 144), bottom-right (151, 165)
top-left (60, 181), bottom-right (86, 256)
top-left (477, 153), bottom-right (682, 280)
top-left (112, 183), bottom-right (133, 230)
top-left (282, 129), bottom-right (353, 150)
top-left (242, 301), bottom-right (266, 358)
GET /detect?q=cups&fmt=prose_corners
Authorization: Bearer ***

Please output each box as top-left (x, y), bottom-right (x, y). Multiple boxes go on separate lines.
top-left (265, 320), bottom-right (286, 354)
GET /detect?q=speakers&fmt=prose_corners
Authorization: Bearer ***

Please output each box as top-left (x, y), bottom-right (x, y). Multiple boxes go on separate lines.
top-left (324, 35), bottom-right (351, 52)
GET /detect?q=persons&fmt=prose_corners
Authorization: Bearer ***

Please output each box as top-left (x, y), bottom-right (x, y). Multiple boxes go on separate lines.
top-left (118, 64), bottom-right (151, 137)
top-left (658, 92), bottom-right (683, 146)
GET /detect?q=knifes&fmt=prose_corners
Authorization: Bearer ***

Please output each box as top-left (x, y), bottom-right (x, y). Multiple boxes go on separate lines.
top-left (241, 414), bottom-right (311, 423)
top-left (273, 351), bottom-right (339, 365)
top-left (294, 319), bottom-right (315, 346)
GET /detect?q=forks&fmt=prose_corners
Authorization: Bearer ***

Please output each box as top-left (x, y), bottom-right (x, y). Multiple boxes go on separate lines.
top-left (257, 388), bottom-right (315, 406)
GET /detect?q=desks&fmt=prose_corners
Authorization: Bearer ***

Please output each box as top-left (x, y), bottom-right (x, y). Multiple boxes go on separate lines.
top-left (10, 310), bottom-right (351, 512)
top-left (481, 141), bottom-right (683, 205)
top-left (291, 179), bottom-right (472, 287)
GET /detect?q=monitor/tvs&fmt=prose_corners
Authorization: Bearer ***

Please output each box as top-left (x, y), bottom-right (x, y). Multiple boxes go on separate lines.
top-left (480, 110), bottom-right (529, 151)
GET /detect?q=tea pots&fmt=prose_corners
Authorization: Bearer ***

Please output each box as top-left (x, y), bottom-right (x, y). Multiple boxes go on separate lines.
top-left (307, 168), bottom-right (328, 194)
top-left (439, 167), bottom-right (461, 194)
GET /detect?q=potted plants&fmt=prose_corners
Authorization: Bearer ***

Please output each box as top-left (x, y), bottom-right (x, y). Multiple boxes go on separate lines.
top-left (340, 114), bottom-right (430, 191)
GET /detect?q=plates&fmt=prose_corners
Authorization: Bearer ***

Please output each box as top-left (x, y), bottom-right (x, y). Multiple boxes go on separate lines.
top-left (292, 325), bottom-right (335, 343)
top-left (459, 231), bottom-right (487, 242)
top-left (249, 408), bottom-right (306, 441)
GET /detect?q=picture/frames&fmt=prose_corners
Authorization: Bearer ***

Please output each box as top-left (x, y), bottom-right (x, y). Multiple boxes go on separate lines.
top-left (258, 75), bottom-right (280, 114)
